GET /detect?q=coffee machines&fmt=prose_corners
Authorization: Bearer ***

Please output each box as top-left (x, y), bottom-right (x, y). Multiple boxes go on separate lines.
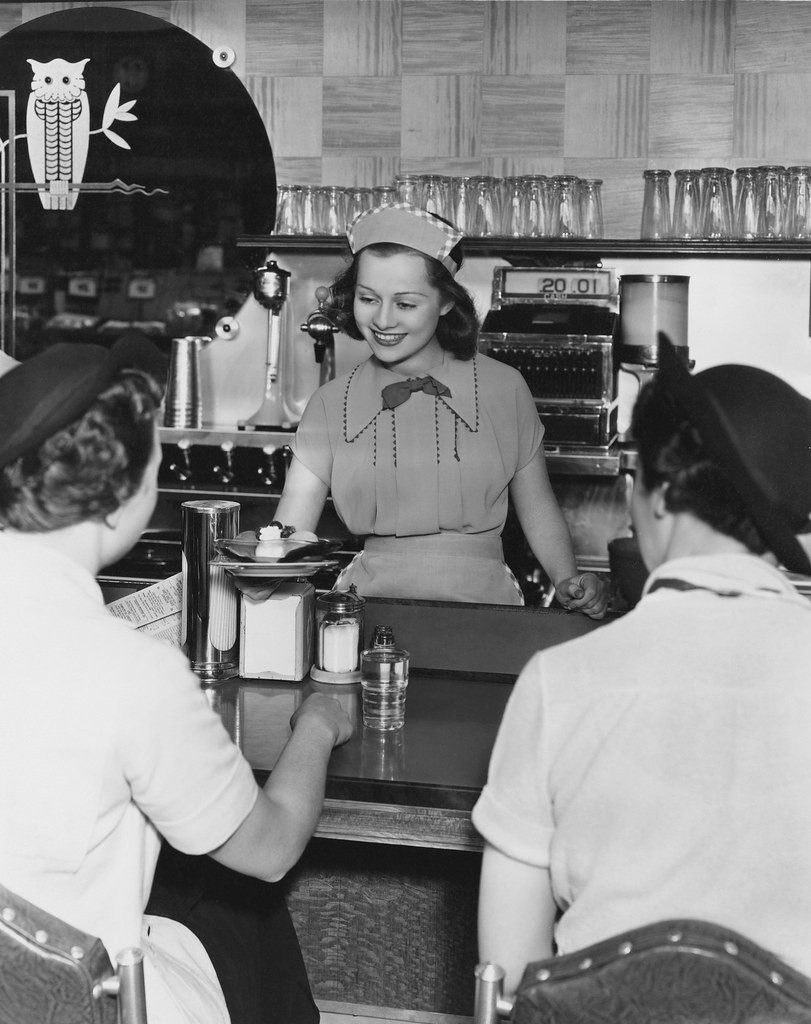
top-left (617, 274), bottom-right (689, 443)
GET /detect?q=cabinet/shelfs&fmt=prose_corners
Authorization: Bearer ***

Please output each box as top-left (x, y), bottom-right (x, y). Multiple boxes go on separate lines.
top-left (149, 230), bottom-right (811, 477)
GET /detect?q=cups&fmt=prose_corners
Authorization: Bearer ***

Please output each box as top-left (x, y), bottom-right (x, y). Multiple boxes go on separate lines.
top-left (639, 169), bottom-right (672, 239)
top-left (395, 175), bottom-right (604, 238)
top-left (164, 339), bottom-right (202, 428)
top-left (735, 165), bottom-right (811, 238)
top-left (672, 167), bottom-right (735, 238)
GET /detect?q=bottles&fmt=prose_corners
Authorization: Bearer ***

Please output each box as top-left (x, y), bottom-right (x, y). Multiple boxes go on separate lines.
top-left (360, 624), bottom-right (411, 693)
top-left (314, 583), bottom-right (366, 673)
top-left (275, 184), bottom-right (397, 235)
top-left (178, 498), bottom-right (240, 679)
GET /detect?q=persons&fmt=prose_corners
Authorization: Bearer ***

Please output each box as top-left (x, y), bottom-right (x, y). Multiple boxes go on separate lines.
top-left (0, 343), bottom-right (353, 1024)
top-left (471, 331), bottom-right (811, 1003)
top-left (271, 205), bottom-right (609, 620)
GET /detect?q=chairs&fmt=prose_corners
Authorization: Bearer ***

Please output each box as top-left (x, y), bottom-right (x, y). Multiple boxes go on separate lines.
top-left (475, 919), bottom-right (811, 1024)
top-left (0, 884), bottom-right (147, 1024)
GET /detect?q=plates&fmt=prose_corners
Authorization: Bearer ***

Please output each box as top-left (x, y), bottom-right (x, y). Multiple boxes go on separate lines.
top-left (209, 560), bottom-right (339, 577)
